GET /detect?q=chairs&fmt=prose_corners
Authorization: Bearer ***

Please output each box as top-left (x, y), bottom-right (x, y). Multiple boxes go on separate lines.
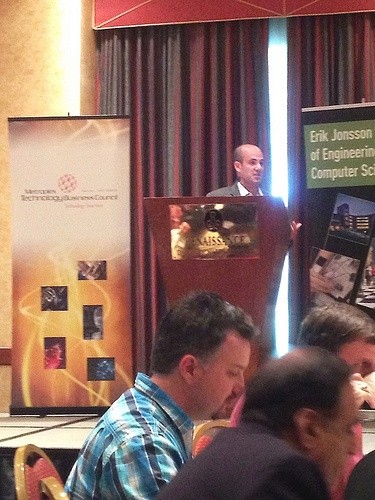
top-left (13, 444), bottom-right (70, 500)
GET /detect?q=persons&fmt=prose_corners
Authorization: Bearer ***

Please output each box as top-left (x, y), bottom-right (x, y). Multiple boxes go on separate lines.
top-left (153, 303), bottom-right (375, 500)
top-left (205, 144), bottom-right (302, 250)
top-left (62, 290), bottom-right (260, 500)
